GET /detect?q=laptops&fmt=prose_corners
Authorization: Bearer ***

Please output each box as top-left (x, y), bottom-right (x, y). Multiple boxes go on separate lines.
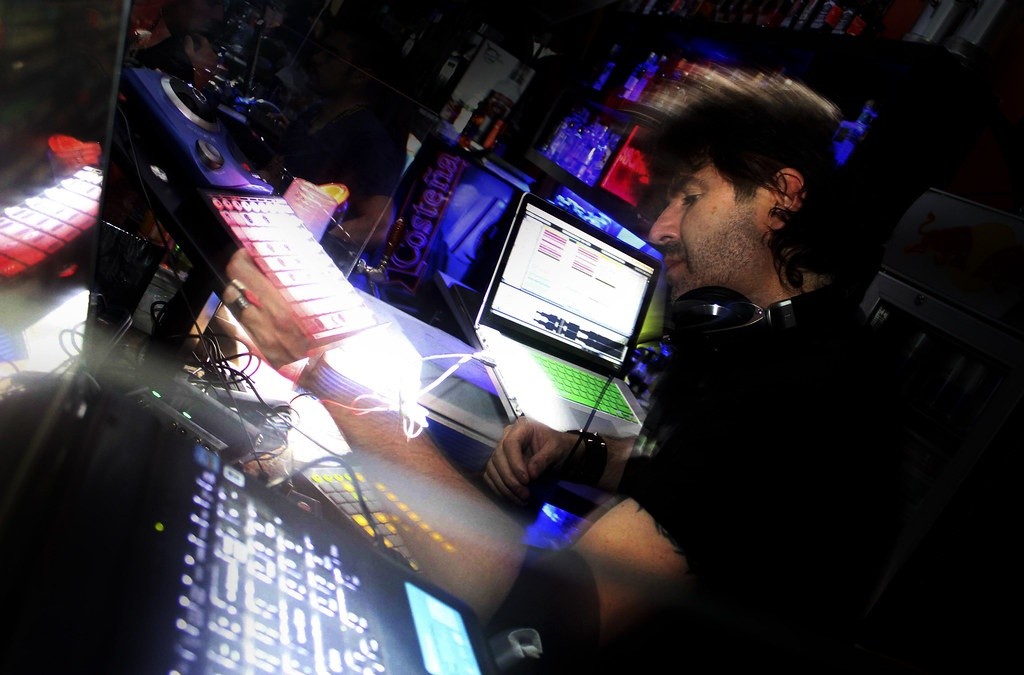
top-left (473, 192), bottom-right (662, 438)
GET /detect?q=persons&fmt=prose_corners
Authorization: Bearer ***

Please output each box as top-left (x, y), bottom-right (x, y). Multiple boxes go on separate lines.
top-left (100, 0), bottom-right (399, 248)
top-left (187, 64), bottom-right (906, 674)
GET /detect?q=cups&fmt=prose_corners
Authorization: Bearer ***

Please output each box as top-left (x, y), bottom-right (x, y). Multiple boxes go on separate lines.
top-left (282, 178), bottom-right (338, 243)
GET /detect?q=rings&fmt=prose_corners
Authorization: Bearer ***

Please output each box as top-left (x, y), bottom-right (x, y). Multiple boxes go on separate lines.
top-left (230, 297), bottom-right (251, 319)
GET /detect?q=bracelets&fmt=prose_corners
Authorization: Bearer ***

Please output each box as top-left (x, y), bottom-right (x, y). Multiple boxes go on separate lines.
top-left (564, 425), bottom-right (610, 485)
top-left (306, 368), bottom-right (342, 402)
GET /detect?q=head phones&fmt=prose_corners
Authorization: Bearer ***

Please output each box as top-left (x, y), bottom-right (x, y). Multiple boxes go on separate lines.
top-left (671, 285), bottom-right (851, 359)
